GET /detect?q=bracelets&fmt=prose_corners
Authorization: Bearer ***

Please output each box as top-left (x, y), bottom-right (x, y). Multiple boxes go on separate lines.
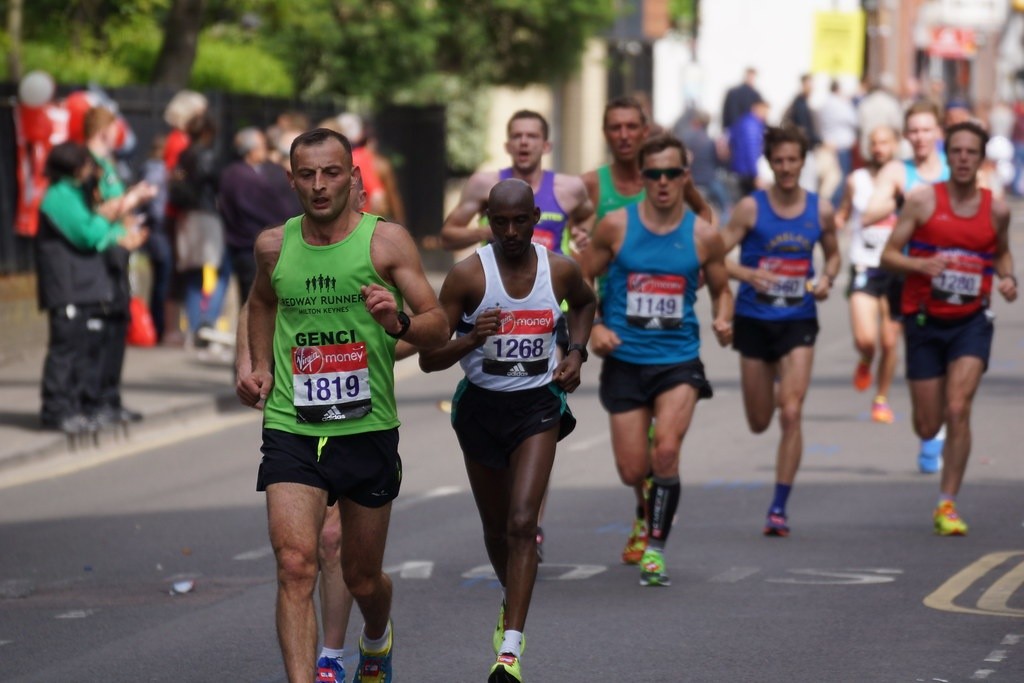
top-left (894, 193), bottom-right (905, 215)
top-left (578, 227), bottom-right (588, 234)
top-left (1001, 273), bottom-right (1017, 287)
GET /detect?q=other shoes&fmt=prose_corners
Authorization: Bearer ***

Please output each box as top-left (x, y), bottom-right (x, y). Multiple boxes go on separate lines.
top-left (56, 405), bottom-right (141, 436)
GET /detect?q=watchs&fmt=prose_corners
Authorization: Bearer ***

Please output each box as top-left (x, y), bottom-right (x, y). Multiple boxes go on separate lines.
top-left (824, 271), bottom-right (834, 287)
top-left (385, 310), bottom-right (410, 339)
top-left (592, 317), bottom-right (604, 326)
top-left (566, 343), bottom-right (588, 362)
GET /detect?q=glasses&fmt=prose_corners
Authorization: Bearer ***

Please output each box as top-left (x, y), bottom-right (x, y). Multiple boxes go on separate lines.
top-left (641, 168), bottom-right (689, 181)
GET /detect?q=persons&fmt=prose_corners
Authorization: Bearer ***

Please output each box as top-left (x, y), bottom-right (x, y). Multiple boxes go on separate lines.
top-left (576, 96), bottom-right (713, 563)
top-left (440, 110), bottom-right (597, 565)
top-left (34, 87), bottom-right (405, 437)
top-left (237, 127), bottom-right (451, 683)
top-left (576, 130), bottom-right (734, 587)
top-left (661, 67), bottom-right (1024, 238)
top-left (861, 99), bottom-right (973, 474)
top-left (236, 161), bottom-right (424, 683)
top-left (719, 120), bottom-right (842, 538)
top-left (880, 121), bottom-right (1019, 536)
top-left (419, 179), bottom-right (597, 683)
top-left (832, 125), bottom-right (904, 424)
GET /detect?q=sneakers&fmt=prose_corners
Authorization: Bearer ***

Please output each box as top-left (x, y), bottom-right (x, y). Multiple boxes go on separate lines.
top-left (853, 358), bottom-right (873, 391)
top-left (487, 650), bottom-right (522, 683)
top-left (536, 526), bottom-right (543, 562)
top-left (932, 499), bottom-right (968, 536)
top-left (353, 616), bottom-right (393, 683)
top-left (492, 596), bottom-right (525, 656)
top-left (638, 548), bottom-right (671, 586)
top-left (871, 397), bottom-right (894, 423)
top-left (917, 436), bottom-right (941, 472)
top-left (314, 656), bottom-right (346, 683)
top-left (762, 505), bottom-right (790, 537)
top-left (623, 517), bottom-right (649, 563)
